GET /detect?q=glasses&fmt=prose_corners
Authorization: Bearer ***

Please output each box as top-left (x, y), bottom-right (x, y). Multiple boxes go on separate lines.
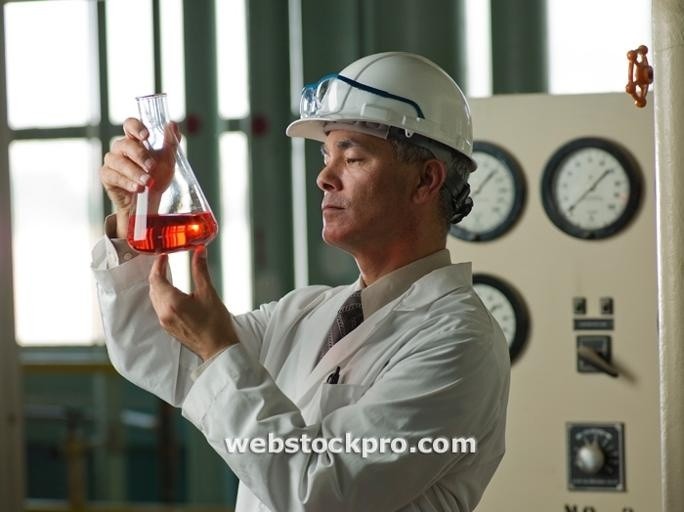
top-left (299, 73), bottom-right (426, 120)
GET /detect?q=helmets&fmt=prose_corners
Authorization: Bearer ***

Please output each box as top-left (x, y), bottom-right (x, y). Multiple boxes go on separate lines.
top-left (285, 51), bottom-right (478, 174)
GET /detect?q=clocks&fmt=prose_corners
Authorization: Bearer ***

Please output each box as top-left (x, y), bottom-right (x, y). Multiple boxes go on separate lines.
top-left (465, 269), bottom-right (530, 367)
top-left (538, 134), bottom-right (646, 242)
top-left (443, 138), bottom-right (529, 244)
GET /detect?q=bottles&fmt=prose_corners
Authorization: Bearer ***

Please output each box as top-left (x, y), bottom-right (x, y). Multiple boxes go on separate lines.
top-left (125, 92), bottom-right (222, 256)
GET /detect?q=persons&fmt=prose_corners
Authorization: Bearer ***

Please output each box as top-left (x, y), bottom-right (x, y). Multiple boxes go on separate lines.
top-left (89, 50), bottom-right (511, 512)
